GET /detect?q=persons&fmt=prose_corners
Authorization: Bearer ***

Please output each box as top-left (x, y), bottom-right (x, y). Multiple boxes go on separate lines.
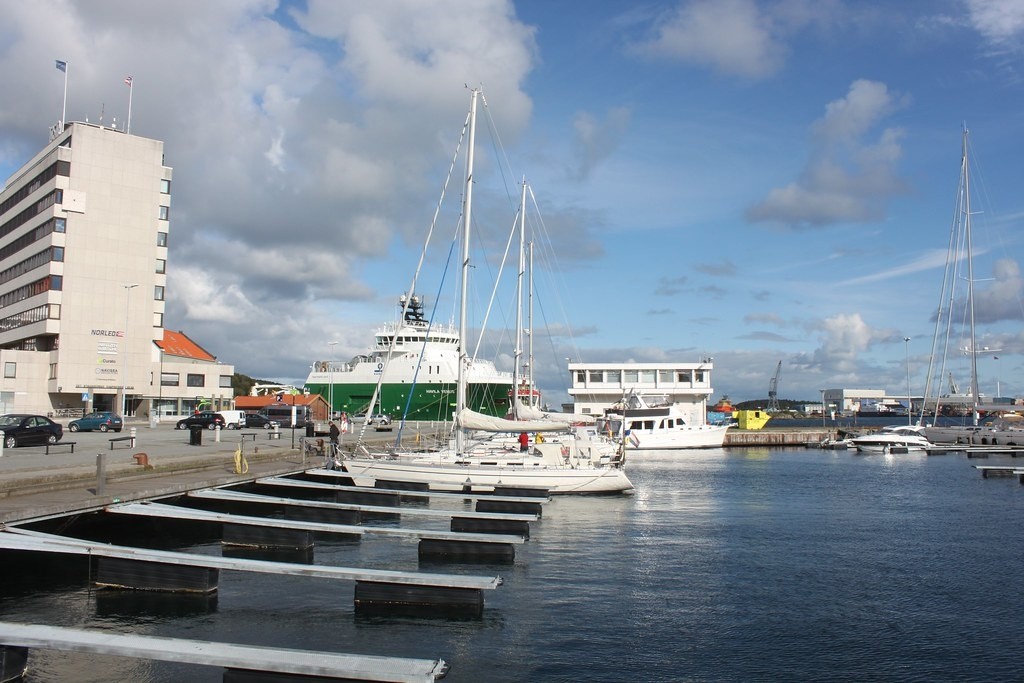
top-left (518, 432), bottom-right (529, 453)
top-left (328, 421), bottom-right (340, 458)
top-left (534, 431), bottom-right (546, 455)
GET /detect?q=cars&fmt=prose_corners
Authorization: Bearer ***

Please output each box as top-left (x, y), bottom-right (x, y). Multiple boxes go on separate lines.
top-left (246, 414), bottom-right (281, 429)
top-left (177, 413), bottom-right (226, 430)
top-left (0, 414), bottom-right (63, 448)
top-left (68, 411), bottom-right (122, 432)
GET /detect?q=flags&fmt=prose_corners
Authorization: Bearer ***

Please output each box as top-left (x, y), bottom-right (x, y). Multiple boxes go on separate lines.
top-left (629, 431), bottom-right (640, 448)
top-left (124, 75), bottom-right (133, 87)
top-left (55, 59), bottom-right (66, 72)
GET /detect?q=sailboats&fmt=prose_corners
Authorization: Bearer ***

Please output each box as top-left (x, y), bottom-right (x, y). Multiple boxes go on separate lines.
top-left (853, 120), bottom-right (1024, 446)
top-left (332, 79), bottom-right (633, 493)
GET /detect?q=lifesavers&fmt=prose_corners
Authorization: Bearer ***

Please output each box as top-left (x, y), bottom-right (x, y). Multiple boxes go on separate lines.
top-left (340, 411), bottom-right (348, 433)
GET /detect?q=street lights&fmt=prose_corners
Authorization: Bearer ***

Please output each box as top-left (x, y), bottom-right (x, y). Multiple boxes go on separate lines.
top-left (903, 337), bottom-right (911, 425)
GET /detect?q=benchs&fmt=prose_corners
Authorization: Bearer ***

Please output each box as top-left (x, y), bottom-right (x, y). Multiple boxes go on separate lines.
top-left (373, 427), bottom-right (393, 432)
top-left (109, 436), bottom-right (136, 450)
top-left (314, 430), bottom-right (330, 437)
top-left (241, 433), bottom-right (257, 441)
top-left (44, 441), bottom-right (76, 454)
top-left (268, 432), bottom-right (282, 439)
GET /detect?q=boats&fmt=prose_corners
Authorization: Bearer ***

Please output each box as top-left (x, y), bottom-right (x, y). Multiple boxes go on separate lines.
top-left (305, 291), bottom-right (535, 426)
top-left (617, 416), bottom-right (727, 449)
top-left (850, 433), bottom-right (936, 454)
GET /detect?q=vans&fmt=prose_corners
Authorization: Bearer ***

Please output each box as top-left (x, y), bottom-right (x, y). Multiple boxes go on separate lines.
top-left (214, 411), bottom-right (246, 430)
top-left (257, 406), bottom-right (297, 428)
top-left (296, 406), bottom-right (308, 429)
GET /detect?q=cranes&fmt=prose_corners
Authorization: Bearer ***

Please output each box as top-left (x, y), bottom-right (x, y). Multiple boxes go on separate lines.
top-left (766, 361), bottom-right (782, 412)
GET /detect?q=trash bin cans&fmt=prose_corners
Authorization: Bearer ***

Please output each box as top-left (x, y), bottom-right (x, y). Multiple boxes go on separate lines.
top-left (189, 425), bottom-right (202, 446)
top-left (306, 422), bottom-right (315, 437)
